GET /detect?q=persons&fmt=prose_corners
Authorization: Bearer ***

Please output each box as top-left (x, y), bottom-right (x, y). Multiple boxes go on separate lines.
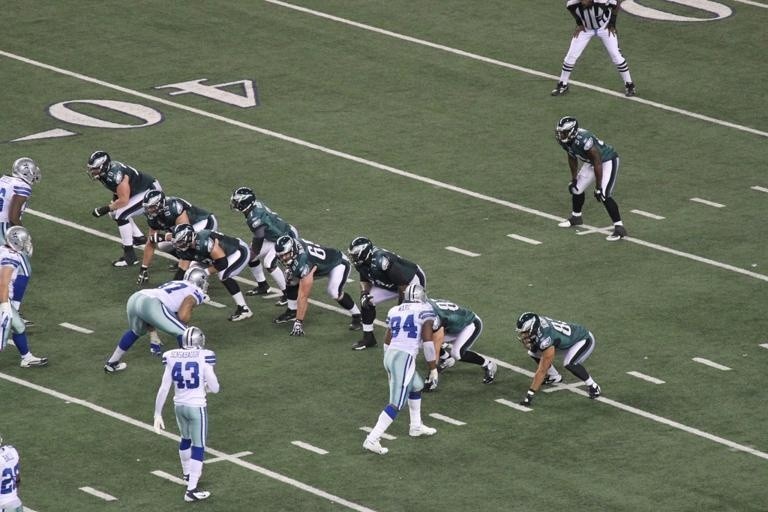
top-left (103, 266), bottom-right (207, 370)
top-left (348, 235), bottom-right (425, 351)
top-left (517, 310), bottom-right (602, 400)
top-left (156, 325), bottom-right (221, 500)
top-left (173, 223), bottom-right (253, 321)
top-left (136, 190), bottom-right (217, 285)
top-left (553, 116), bottom-right (627, 242)
top-left (423, 296), bottom-right (497, 390)
top-left (1, 227), bottom-right (51, 368)
top-left (364, 285), bottom-right (438, 456)
top-left (272, 234), bottom-right (362, 336)
top-left (0, 158), bottom-right (40, 332)
top-left (551, 1), bottom-right (638, 95)
top-left (87, 152), bottom-right (162, 266)
top-left (230, 187), bottom-right (298, 306)
top-left (0, 435), bottom-right (24, 512)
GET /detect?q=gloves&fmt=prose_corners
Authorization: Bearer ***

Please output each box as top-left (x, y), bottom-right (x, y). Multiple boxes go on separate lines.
top-left (149, 331), bottom-right (163, 357)
top-left (153, 415), bottom-right (166, 435)
top-left (567, 179), bottom-right (579, 195)
top-left (592, 186), bottom-right (606, 204)
top-left (136, 266), bottom-right (149, 285)
top-left (519, 388), bottom-right (536, 406)
top-left (0, 300), bottom-right (12, 330)
top-left (289, 319), bottom-right (304, 336)
top-left (92, 205), bottom-right (112, 218)
top-left (424, 368), bottom-right (439, 392)
top-left (150, 232), bottom-right (170, 245)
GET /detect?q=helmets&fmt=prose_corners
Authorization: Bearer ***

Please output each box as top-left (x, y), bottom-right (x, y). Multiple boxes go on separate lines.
top-left (4, 224), bottom-right (34, 258)
top-left (12, 156), bottom-right (43, 186)
top-left (348, 235), bottom-right (374, 266)
top-left (86, 150), bottom-right (112, 182)
top-left (273, 234), bottom-right (298, 269)
top-left (181, 325), bottom-right (206, 350)
top-left (554, 115), bottom-right (580, 145)
top-left (229, 187), bottom-right (256, 215)
top-left (172, 223), bottom-right (197, 255)
top-left (515, 312), bottom-right (540, 347)
top-left (183, 267), bottom-right (209, 290)
top-left (404, 283), bottom-right (427, 304)
top-left (142, 189), bottom-right (166, 222)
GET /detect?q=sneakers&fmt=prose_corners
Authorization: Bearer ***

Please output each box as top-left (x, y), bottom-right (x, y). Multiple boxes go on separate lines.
top-left (182, 474), bottom-right (211, 503)
top-left (20, 357), bottom-right (48, 369)
top-left (348, 312), bottom-right (364, 332)
top-left (481, 360), bottom-right (498, 385)
top-left (351, 331), bottom-right (379, 350)
top-left (229, 280), bottom-right (299, 325)
top-left (557, 215), bottom-right (629, 243)
top-left (551, 80), bottom-right (639, 96)
top-left (437, 354), bottom-right (457, 373)
top-left (361, 434), bottom-right (389, 456)
top-left (17, 311), bottom-right (36, 330)
top-left (104, 361), bottom-right (128, 375)
top-left (409, 423), bottom-right (438, 438)
top-left (541, 374), bottom-right (603, 401)
top-left (112, 236), bottom-right (148, 268)
top-left (167, 263), bottom-right (179, 273)
